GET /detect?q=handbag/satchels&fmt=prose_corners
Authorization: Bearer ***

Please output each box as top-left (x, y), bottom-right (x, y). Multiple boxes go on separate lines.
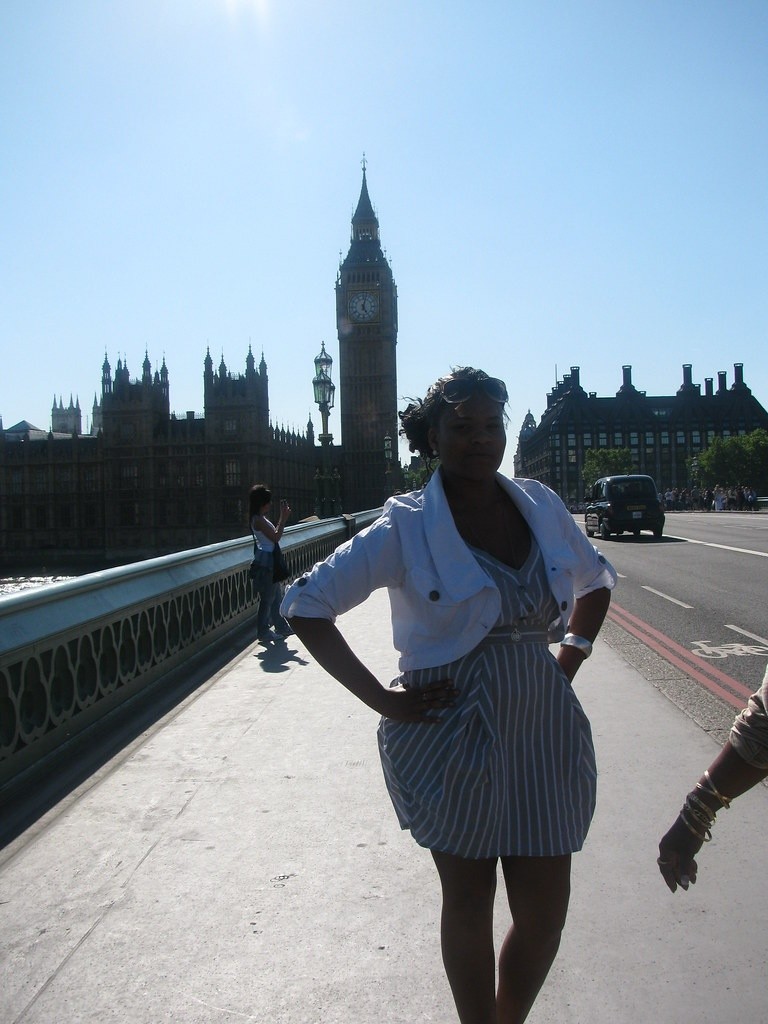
top-left (271, 542), bottom-right (290, 583)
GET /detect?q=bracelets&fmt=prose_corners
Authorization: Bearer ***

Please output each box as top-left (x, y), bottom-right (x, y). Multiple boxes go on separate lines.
top-left (561, 633), bottom-right (592, 659)
top-left (680, 809), bottom-right (712, 842)
top-left (704, 770), bottom-right (731, 809)
top-left (696, 783), bottom-right (733, 802)
top-left (684, 793), bottom-right (716, 829)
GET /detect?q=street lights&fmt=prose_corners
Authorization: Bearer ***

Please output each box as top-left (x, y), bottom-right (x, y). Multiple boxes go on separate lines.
top-left (312, 341), bottom-right (342, 518)
top-left (383, 431), bottom-right (394, 496)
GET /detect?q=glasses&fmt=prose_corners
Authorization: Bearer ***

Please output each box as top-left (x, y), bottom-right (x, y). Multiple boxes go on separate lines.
top-left (431, 377), bottom-right (508, 422)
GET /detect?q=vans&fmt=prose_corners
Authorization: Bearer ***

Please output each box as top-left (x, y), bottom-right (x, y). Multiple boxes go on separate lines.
top-left (585, 475), bottom-right (665, 539)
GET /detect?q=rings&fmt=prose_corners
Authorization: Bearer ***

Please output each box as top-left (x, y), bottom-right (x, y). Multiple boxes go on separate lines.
top-left (656, 857), bottom-right (672, 865)
top-left (423, 692), bottom-right (427, 700)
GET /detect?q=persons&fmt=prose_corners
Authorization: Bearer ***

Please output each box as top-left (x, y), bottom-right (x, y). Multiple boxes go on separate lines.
top-left (393, 483), bottom-right (427, 495)
top-left (277, 366), bottom-right (618, 1024)
top-left (248, 484), bottom-right (292, 640)
top-left (570, 484), bottom-right (758, 513)
top-left (658, 665), bottom-right (768, 894)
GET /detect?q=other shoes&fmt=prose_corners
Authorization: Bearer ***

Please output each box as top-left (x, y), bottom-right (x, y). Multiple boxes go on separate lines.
top-left (258, 630), bottom-right (283, 641)
top-left (275, 626), bottom-right (295, 636)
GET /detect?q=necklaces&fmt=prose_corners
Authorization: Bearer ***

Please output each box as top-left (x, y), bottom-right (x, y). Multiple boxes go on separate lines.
top-left (448, 481), bottom-right (517, 571)
